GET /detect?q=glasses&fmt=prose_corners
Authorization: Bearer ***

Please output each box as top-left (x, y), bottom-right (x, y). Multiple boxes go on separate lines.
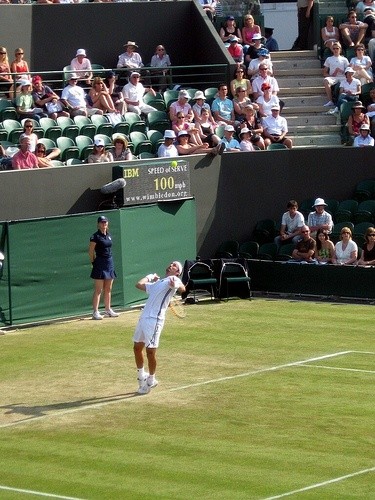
top-left (351, 16), bottom-right (357, 18)
top-left (367, 234), bottom-right (375, 236)
top-left (302, 230), bottom-right (308, 233)
top-left (357, 49), bottom-right (363, 51)
top-left (260, 68), bottom-right (267, 70)
top-left (17, 53), bottom-right (24, 55)
top-left (25, 125), bottom-right (33, 128)
top-left (157, 49), bottom-right (165, 51)
top-left (178, 117), bottom-right (184, 119)
top-left (0, 52), bottom-right (6, 54)
top-left (236, 71), bottom-right (243, 73)
top-left (332, 48), bottom-right (339, 50)
top-left (37, 150), bottom-right (44, 153)
top-left (342, 232), bottom-right (349, 234)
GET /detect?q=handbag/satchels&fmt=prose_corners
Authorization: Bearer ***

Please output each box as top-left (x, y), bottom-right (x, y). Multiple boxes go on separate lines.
top-left (44, 102), bottom-right (62, 115)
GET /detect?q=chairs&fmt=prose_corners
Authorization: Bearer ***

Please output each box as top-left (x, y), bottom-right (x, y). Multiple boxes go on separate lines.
top-left (186, 258), bottom-right (217, 303)
top-left (266, 143), bottom-right (289, 151)
top-left (340, 101), bottom-right (360, 146)
top-left (221, 258), bottom-right (251, 300)
top-left (205, 88), bottom-right (220, 100)
top-left (323, 47), bottom-right (344, 62)
top-left (346, 45), bottom-right (367, 62)
top-left (167, 97), bottom-right (179, 113)
top-left (361, 82), bottom-right (375, 108)
top-left (334, 83), bottom-right (339, 103)
top-left (62, 65), bottom-right (73, 85)
top-left (91, 63), bottom-right (104, 80)
top-left (216, 177), bottom-right (375, 258)
top-left (186, 89), bottom-right (202, 101)
top-left (216, 126), bottom-right (237, 139)
top-left (164, 90), bottom-right (183, 109)
top-left (0, 90), bottom-right (171, 165)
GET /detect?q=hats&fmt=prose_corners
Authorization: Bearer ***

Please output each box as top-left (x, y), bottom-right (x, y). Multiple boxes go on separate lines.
top-left (164, 130), bottom-right (176, 139)
top-left (179, 90), bottom-right (191, 99)
top-left (239, 128), bottom-right (254, 139)
top-left (351, 101), bottom-right (366, 109)
top-left (230, 36), bottom-right (238, 42)
top-left (123, 41), bottom-right (139, 48)
top-left (32, 76), bottom-right (41, 84)
top-left (111, 135), bottom-right (128, 148)
top-left (192, 91), bottom-right (206, 100)
top-left (224, 125), bottom-right (235, 132)
top-left (345, 67), bottom-right (356, 74)
top-left (261, 83), bottom-right (272, 90)
top-left (76, 49), bottom-right (87, 58)
top-left (98, 216), bottom-right (108, 222)
top-left (130, 72), bottom-right (141, 77)
top-left (177, 130), bottom-right (191, 139)
top-left (251, 33), bottom-right (264, 42)
top-left (359, 124), bottom-right (369, 130)
top-left (257, 47), bottom-right (270, 59)
top-left (95, 138), bottom-right (104, 146)
top-left (20, 80), bottom-right (31, 91)
top-left (226, 15), bottom-right (235, 20)
top-left (271, 104), bottom-right (280, 111)
top-left (66, 73), bottom-right (80, 81)
top-left (312, 198), bottom-right (328, 208)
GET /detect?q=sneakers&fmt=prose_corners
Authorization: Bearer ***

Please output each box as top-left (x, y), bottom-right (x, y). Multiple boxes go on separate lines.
top-left (92, 313), bottom-right (103, 320)
top-left (136, 373), bottom-right (149, 392)
top-left (139, 379), bottom-right (158, 395)
top-left (105, 310), bottom-right (118, 317)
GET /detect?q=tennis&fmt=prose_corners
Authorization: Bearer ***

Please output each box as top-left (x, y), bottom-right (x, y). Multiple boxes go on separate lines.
top-left (170, 161), bottom-right (178, 168)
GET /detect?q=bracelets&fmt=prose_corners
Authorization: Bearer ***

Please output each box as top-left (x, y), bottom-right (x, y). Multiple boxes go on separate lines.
top-left (146, 274), bottom-right (154, 281)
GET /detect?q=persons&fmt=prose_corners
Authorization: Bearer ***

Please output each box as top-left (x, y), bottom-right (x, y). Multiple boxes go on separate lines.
top-left (326, 67), bottom-right (362, 115)
top-left (34, 143), bottom-right (61, 168)
top-left (274, 200), bottom-right (305, 248)
top-left (19, 120), bottom-right (38, 153)
top-left (251, 64), bottom-right (279, 97)
top-left (296, 0), bottom-right (314, 50)
top-left (221, 125), bottom-right (241, 151)
top-left (133, 261), bottom-right (186, 394)
top-left (117, 41), bottom-right (145, 72)
top-left (334, 227), bottom-right (358, 265)
top-left (69, 49), bottom-right (93, 87)
top-left (292, 225), bottom-right (316, 260)
top-left (88, 138), bottom-right (113, 162)
top-left (354, 0), bottom-right (375, 39)
top-left (230, 67), bottom-right (253, 98)
top-left (255, 83), bottom-right (280, 118)
top-left (220, 15), bottom-right (243, 49)
top-left (103, 71), bottom-right (127, 116)
top-left (169, 90), bottom-right (195, 123)
top-left (0, 143), bottom-right (13, 170)
top-left (261, 104), bottom-right (292, 149)
top-left (199, 0), bottom-right (216, 22)
top-left (236, 104), bottom-right (266, 150)
top-left (122, 72), bottom-right (158, 115)
top-left (241, 14), bottom-right (261, 51)
top-left (172, 111), bottom-right (203, 145)
top-left (32, 75), bottom-right (70, 119)
top-left (365, 88), bottom-right (375, 139)
top-left (245, 34), bottom-right (270, 63)
top-left (88, 216), bottom-right (119, 320)
top-left (177, 130), bottom-right (226, 155)
top-left (233, 86), bottom-right (259, 122)
top-left (14, 80), bottom-right (48, 122)
top-left (12, 135), bottom-right (39, 171)
top-left (61, 73), bottom-right (103, 120)
top-left (356, 227), bottom-right (375, 265)
top-left (322, 42), bottom-right (349, 106)
top-left (238, 128), bottom-right (255, 151)
top-left (195, 108), bottom-right (222, 146)
top-left (350, 44), bottom-right (374, 86)
top-left (247, 49), bottom-right (273, 81)
top-left (314, 228), bottom-right (335, 262)
top-left (353, 124), bottom-right (374, 147)
top-left (0, 47), bottom-right (13, 99)
top-left (348, 101), bottom-right (370, 140)
top-left (212, 84), bottom-right (240, 126)
top-left (88, 77), bottom-right (120, 114)
top-left (112, 135), bottom-right (131, 160)
top-left (339, 11), bottom-right (368, 46)
top-left (320, 16), bottom-right (340, 68)
top-left (158, 130), bottom-right (179, 157)
top-left (150, 45), bottom-right (172, 95)
top-left (266, 27), bottom-right (278, 50)
top-left (227, 37), bottom-right (247, 69)
top-left (10, 48), bottom-right (33, 91)
top-left (307, 198), bottom-right (334, 239)
top-left (192, 91), bottom-right (217, 126)
